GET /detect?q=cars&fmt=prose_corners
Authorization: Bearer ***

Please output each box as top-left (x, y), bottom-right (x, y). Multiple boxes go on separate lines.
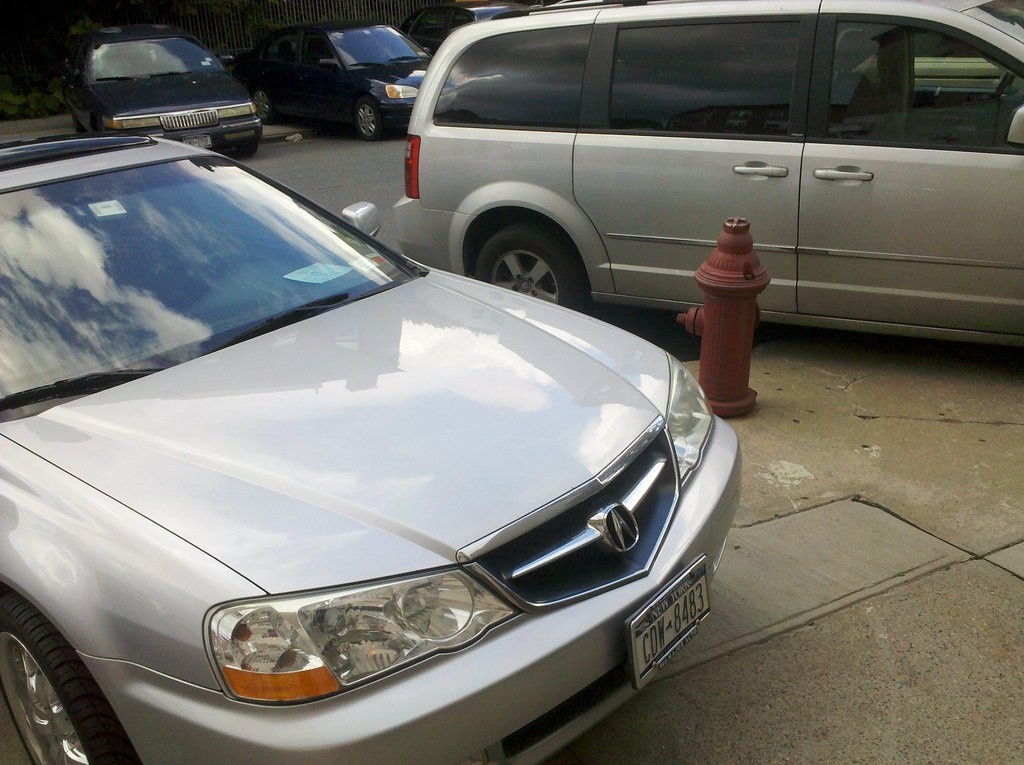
top-left (62, 21), bottom-right (263, 159)
top-left (231, 24), bottom-right (456, 141)
top-left (0, 133), bottom-right (744, 765)
top-left (397, 3), bottom-right (530, 63)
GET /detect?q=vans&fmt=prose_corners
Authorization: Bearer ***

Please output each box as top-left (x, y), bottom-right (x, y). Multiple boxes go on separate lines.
top-left (386, 1), bottom-right (1022, 356)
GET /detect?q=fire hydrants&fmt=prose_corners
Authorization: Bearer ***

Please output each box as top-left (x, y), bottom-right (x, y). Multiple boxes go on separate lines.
top-left (667, 217), bottom-right (776, 415)
